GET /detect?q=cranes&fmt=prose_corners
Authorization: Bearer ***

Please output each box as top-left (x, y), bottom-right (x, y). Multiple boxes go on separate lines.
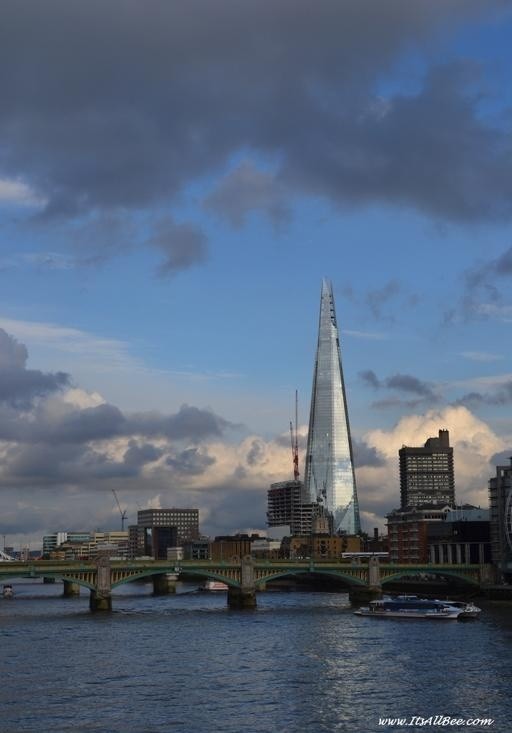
top-left (289, 388), bottom-right (300, 479)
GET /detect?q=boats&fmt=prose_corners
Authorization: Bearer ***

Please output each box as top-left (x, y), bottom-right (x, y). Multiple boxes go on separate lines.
top-left (198, 577), bottom-right (228, 594)
top-left (354, 596), bottom-right (482, 620)
top-left (2, 583), bottom-right (14, 599)
